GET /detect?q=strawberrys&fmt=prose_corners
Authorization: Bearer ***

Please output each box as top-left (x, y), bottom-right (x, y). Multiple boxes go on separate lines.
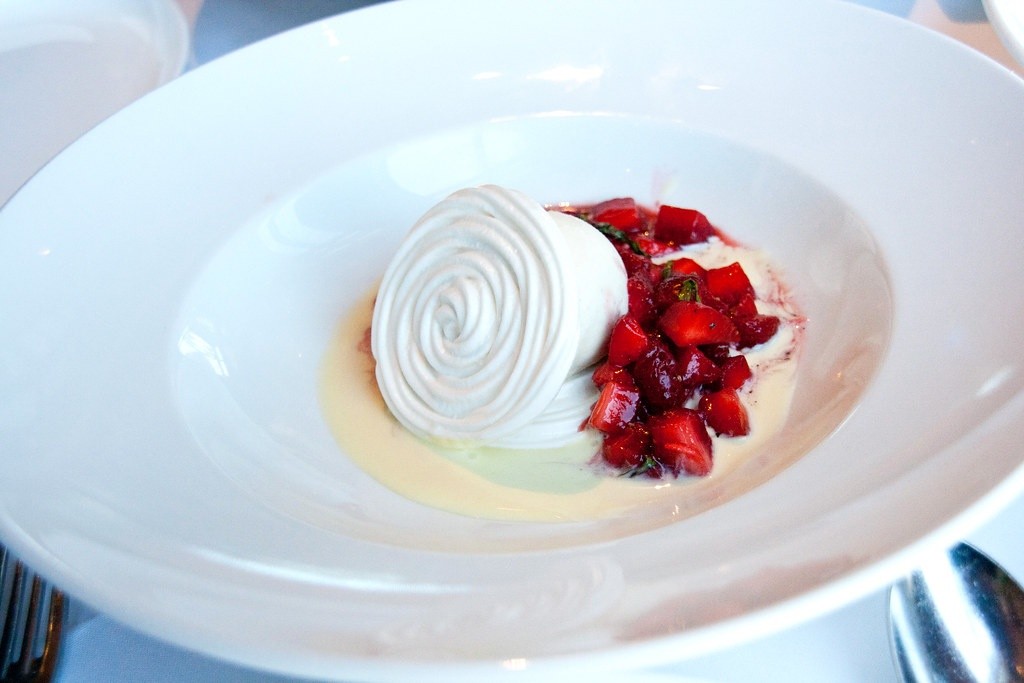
top-left (557, 196), bottom-right (782, 479)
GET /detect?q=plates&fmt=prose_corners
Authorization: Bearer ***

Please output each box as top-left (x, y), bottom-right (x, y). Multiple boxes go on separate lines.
top-left (0, 0), bottom-right (1024, 683)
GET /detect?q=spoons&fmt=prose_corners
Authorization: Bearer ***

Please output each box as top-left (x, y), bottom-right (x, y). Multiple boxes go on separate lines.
top-left (887, 540), bottom-right (1024, 683)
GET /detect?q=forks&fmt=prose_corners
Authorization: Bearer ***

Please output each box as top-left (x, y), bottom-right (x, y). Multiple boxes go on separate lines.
top-left (0, 545), bottom-right (63, 683)
